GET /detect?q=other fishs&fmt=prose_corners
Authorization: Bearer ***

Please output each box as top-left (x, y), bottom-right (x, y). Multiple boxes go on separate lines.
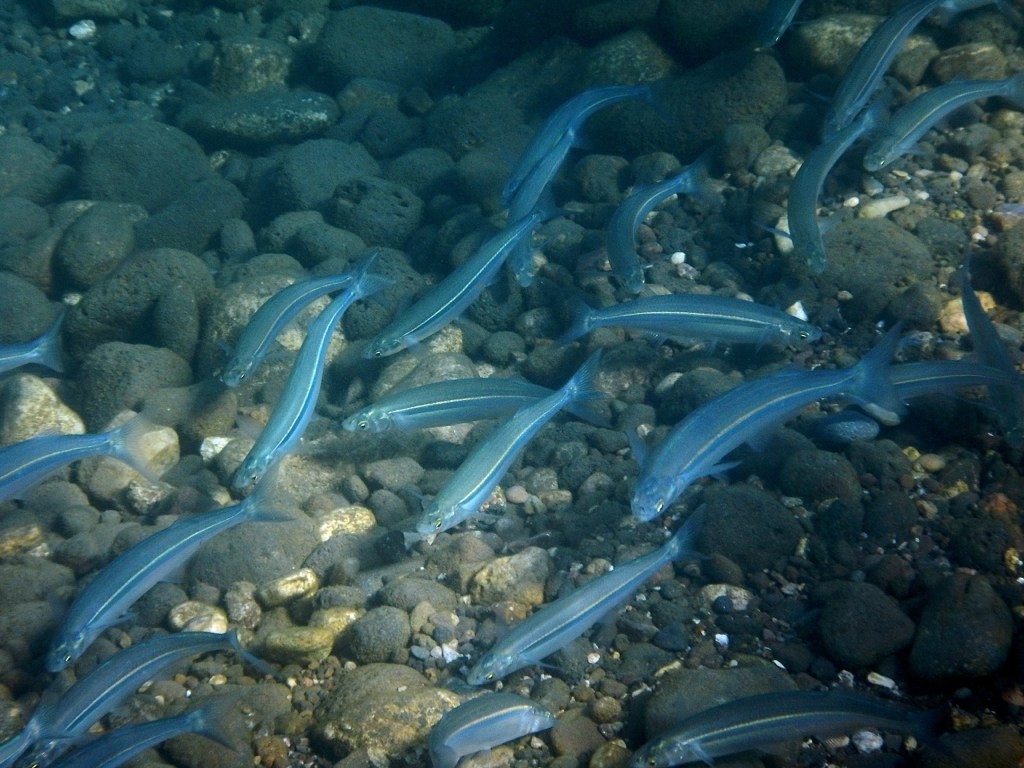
top-left (0, 0), bottom-right (1024, 768)
